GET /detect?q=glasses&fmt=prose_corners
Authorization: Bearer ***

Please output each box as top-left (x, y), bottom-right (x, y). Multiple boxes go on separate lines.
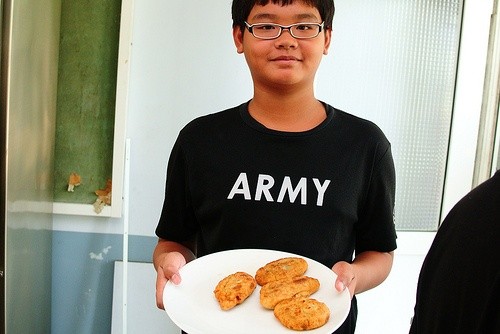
top-left (244, 21), bottom-right (324, 40)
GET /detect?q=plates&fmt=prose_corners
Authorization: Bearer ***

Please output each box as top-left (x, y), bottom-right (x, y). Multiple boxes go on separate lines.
top-left (162, 249), bottom-right (351, 334)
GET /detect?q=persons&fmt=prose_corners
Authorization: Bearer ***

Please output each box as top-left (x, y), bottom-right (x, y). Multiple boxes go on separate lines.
top-left (152, 0), bottom-right (398, 334)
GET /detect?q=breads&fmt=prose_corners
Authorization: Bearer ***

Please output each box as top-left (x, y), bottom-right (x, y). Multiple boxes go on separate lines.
top-left (255, 257), bottom-right (308, 286)
top-left (273, 297), bottom-right (330, 331)
top-left (214, 272), bottom-right (257, 310)
top-left (260, 276), bottom-right (320, 310)
